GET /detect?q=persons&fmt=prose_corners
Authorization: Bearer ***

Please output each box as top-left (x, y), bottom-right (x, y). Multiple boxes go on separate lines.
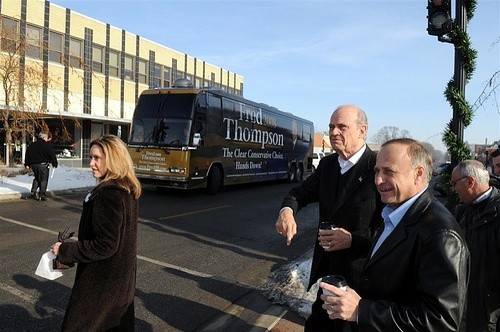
top-left (276, 105), bottom-right (500, 332)
top-left (24, 130), bottom-right (59, 201)
top-left (35, 135), bottom-right (141, 332)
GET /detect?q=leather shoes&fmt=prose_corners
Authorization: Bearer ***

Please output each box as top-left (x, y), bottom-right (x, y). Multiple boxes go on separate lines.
top-left (28, 192), bottom-right (47, 201)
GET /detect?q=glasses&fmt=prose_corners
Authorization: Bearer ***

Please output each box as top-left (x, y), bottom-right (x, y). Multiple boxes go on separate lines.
top-left (448, 176), bottom-right (469, 187)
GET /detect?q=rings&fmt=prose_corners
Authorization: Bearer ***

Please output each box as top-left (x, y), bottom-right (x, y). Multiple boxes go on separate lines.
top-left (50, 249), bottom-right (53, 252)
top-left (332, 305), bottom-right (335, 311)
top-left (328, 242), bottom-right (331, 245)
top-left (279, 232), bottom-right (282, 235)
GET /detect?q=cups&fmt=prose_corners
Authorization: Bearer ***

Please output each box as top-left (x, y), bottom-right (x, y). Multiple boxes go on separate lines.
top-left (322, 276), bottom-right (347, 315)
top-left (319, 222), bottom-right (335, 249)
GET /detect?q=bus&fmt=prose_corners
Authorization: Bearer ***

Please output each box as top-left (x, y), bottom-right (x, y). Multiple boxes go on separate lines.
top-left (127, 78), bottom-right (315, 197)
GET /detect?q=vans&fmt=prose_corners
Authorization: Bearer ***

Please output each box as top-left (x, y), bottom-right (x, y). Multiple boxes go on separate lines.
top-left (312, 152), bottom-right (325, 174)
